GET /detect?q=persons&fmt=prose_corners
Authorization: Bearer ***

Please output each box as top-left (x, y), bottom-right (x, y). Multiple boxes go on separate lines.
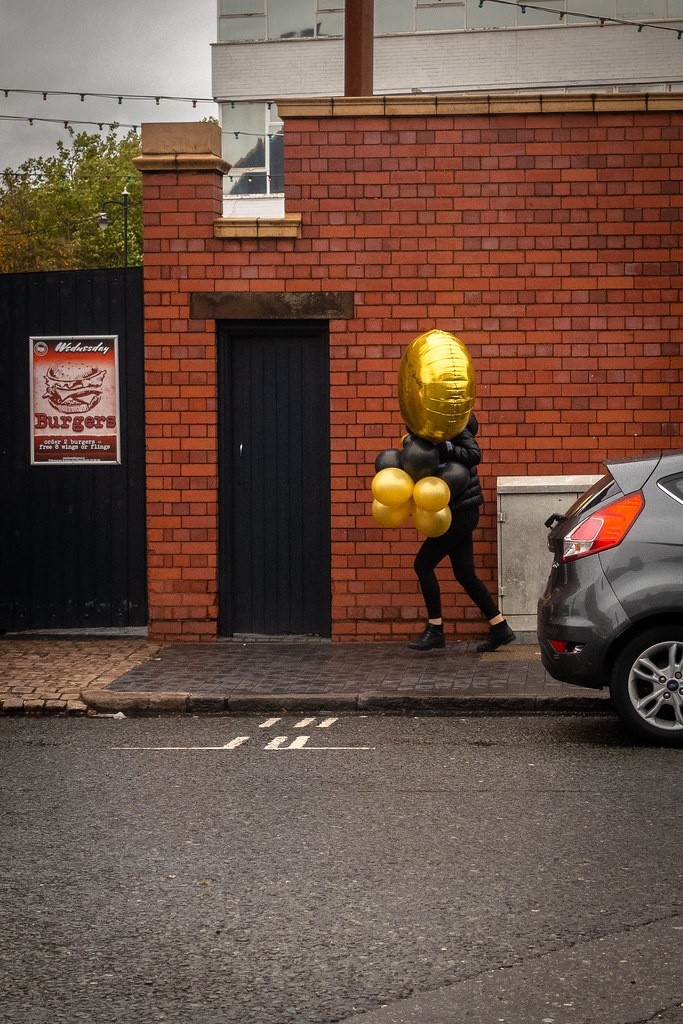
top-left (406, 430), bottom-right (517, 653)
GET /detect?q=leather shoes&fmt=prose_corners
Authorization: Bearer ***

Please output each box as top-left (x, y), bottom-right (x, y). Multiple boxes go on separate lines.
top-left (407, 622), bottom-right (445, 651)
top-left (477, 619), bottom-right (516, 652)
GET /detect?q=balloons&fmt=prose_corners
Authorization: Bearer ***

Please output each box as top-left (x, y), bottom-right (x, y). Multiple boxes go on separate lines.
top-left (371, 329), bottom-right (473, 537)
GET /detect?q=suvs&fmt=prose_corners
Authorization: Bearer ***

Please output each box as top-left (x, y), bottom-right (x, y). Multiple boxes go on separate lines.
top-left (533, 450), bottom-right (683, 748)
